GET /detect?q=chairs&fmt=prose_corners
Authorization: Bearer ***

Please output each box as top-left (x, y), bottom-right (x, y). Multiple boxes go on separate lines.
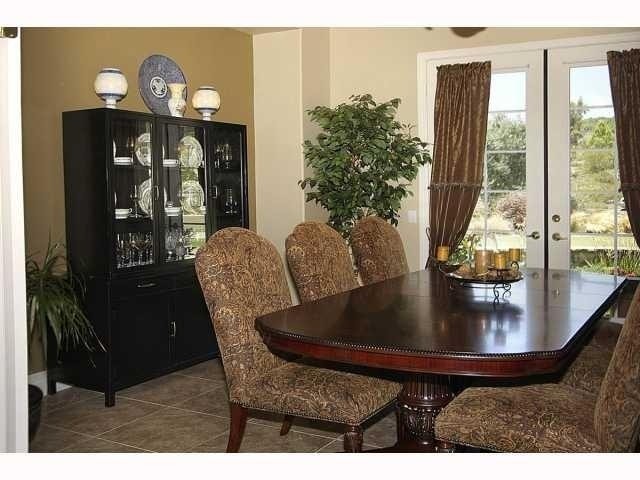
top-left (284, 219), bottom-right (363, 305)
top-left (194, 226), bottom-right (405, 453)
top-left (350, 214), bottom-right (411, 288)
top-left (434, 272), bottom-right (639, 452)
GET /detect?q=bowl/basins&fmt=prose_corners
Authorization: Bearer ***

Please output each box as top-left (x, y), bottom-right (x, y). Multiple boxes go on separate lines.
top-left (115, 209), bottom-right (129, 219)
top-left (163, 159), bottom-right (177, 166)
top-left (165, 206), bottom-right (179, 217)
top-left (114, 158), bottom-right (132, 166)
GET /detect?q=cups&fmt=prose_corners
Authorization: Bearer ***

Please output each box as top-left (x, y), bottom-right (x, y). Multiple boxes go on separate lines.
top-left (435, 245), bottom-right (520, 275)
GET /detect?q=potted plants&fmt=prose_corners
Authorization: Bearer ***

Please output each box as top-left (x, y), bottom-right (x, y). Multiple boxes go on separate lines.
top-left (26, 216), bottom-right (107, 450)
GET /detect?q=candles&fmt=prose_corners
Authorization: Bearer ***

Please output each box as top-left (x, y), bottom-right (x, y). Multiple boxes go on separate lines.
top-left (433, 240), bottom-right (527, 275)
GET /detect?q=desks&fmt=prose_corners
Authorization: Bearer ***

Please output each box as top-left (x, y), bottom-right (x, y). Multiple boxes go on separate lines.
top-left (250, 265), bottom-right (629, 452)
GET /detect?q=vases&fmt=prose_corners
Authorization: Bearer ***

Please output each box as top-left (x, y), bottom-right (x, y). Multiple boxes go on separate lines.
top-left (190, 85), bottom-right (222, 123)
top-left (92, 66), bottom-right (129, 109)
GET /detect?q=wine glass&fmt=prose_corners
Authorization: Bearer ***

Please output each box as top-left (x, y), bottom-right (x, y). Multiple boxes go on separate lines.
top-left (223, 187), bottom-right (240, 215)
top-left (114, 229), bottom-right (192, 267)
top-left (213, 143), bottom-right (232, 169)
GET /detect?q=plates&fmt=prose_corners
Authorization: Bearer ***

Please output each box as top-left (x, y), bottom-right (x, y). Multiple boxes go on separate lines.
top-left (136, 133), bottom-right (165, 164)
top-left (136, 53), bottom-right (187, 115)
top-left (177, 134), bottom-right (204, 168)
top-left (179, 181), bottom-right (205, 211)
top-left (137, 178), bottom-right (168, 216)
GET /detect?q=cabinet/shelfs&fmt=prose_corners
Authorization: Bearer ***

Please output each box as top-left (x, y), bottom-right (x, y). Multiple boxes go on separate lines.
top-left (43, 106), bottom-right (249, 412)
